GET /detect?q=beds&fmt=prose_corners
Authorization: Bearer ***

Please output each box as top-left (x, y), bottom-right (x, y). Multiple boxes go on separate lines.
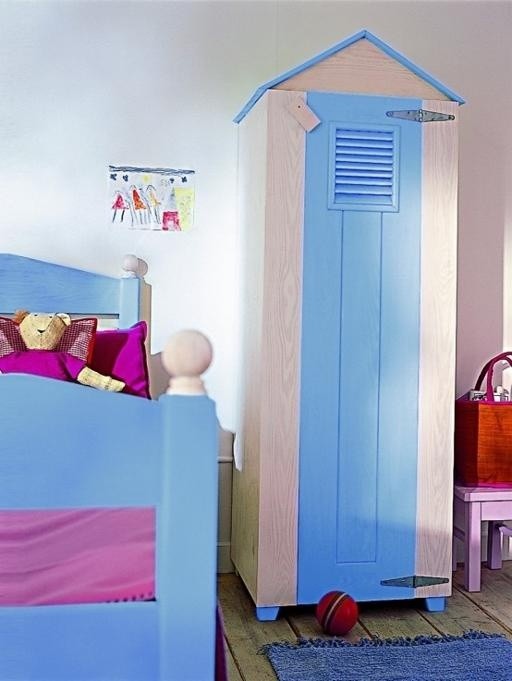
top-left (0, 252), bottom-right (219, 680)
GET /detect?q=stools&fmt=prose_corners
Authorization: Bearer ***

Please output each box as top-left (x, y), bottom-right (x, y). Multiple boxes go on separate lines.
top-left (452, 486), bottom-right (512, 592)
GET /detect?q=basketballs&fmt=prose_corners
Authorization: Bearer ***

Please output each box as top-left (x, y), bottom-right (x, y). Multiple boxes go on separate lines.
top-left (317, 591), bottom-right (358, 634)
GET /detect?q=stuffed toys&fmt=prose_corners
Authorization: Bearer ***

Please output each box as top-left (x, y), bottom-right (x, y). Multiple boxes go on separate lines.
top-left (0, 309), bottom-right (126, 393)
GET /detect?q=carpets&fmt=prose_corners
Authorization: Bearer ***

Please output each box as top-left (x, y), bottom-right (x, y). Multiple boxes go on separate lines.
top-left (257, 629), bottom-right (512, 681)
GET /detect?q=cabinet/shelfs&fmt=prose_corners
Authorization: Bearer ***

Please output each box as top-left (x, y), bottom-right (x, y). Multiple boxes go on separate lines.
top-left (231, 28), bottom-right (467, 622)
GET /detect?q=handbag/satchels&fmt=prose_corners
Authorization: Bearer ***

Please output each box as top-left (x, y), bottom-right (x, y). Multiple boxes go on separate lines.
top-left (455, 351), bottom-right (512, 488)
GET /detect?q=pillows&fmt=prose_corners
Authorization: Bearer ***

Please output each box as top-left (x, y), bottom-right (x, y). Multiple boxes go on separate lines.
top-left (88, 321), bottom-right (152, 400)
top-left (0, 317), bottom-right (97, 368)
